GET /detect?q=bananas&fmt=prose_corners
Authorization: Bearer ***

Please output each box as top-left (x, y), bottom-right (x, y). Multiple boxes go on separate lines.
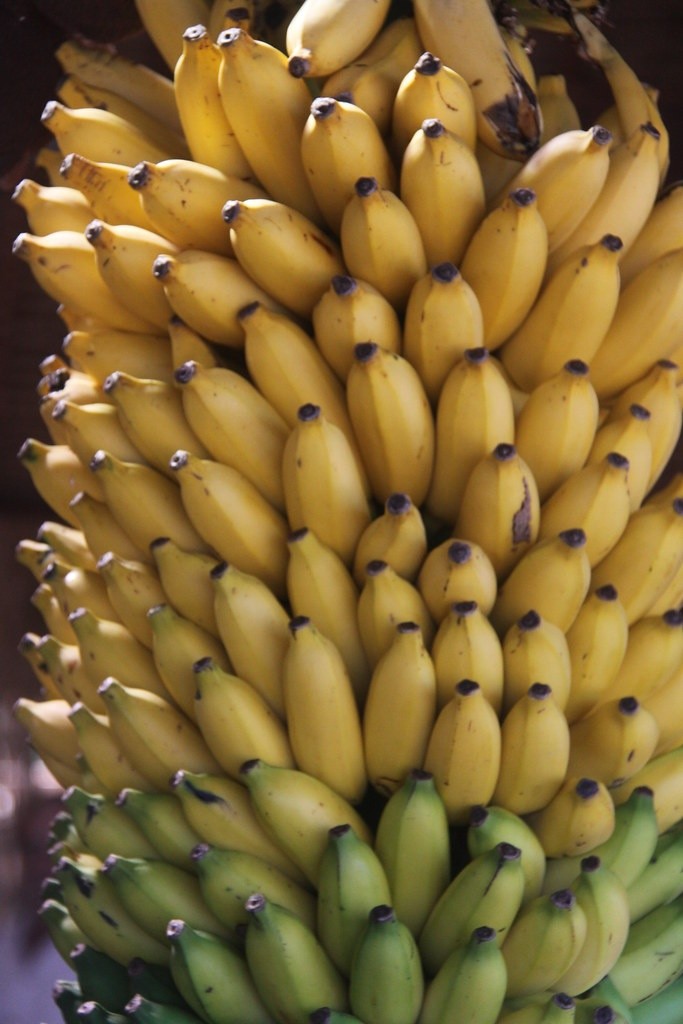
top-left (10, 0), bottom-right (682, 1024)
